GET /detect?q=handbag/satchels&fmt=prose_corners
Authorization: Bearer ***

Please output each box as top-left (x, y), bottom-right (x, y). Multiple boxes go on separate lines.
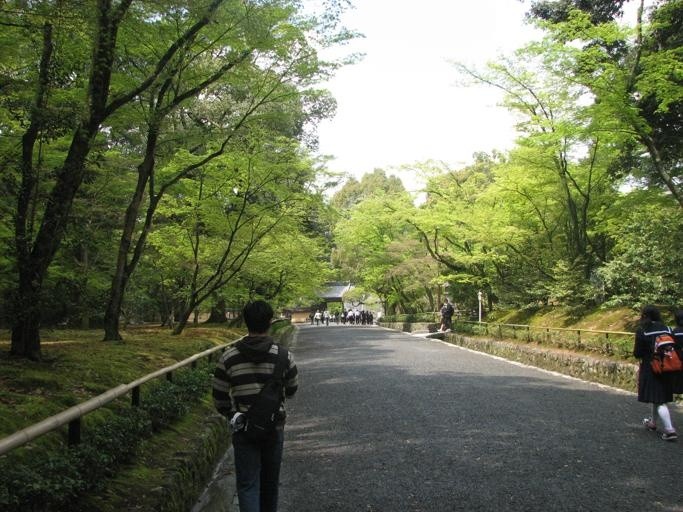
top-left (226, 342), bottom-right (286, 448)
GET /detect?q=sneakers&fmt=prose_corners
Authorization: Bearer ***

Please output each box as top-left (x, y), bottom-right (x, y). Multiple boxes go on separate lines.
top-left (640, 417), bottom-right (657, 433)
top-left (660, 430), bottom-right (677, 441)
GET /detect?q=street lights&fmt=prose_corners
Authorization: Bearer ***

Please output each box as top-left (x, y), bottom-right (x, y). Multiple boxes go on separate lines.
top-left (477, 291), bottom-right (484, 323)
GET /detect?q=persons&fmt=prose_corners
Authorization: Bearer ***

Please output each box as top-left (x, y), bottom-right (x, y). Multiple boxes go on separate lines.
top-left (670, 312), bottom-right (682, 397)
top-left (209, 298), bottom-right (299, 512)
top-left (307, 306), bottom-right (375, 327)
top-left (630, 303), bottom-right (682, 441)
top-left (439, 298), bottom-right (453, 333)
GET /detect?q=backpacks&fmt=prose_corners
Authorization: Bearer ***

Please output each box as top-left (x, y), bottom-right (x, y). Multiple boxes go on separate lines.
top-left (648, 333), bottom-right (682, 376)
